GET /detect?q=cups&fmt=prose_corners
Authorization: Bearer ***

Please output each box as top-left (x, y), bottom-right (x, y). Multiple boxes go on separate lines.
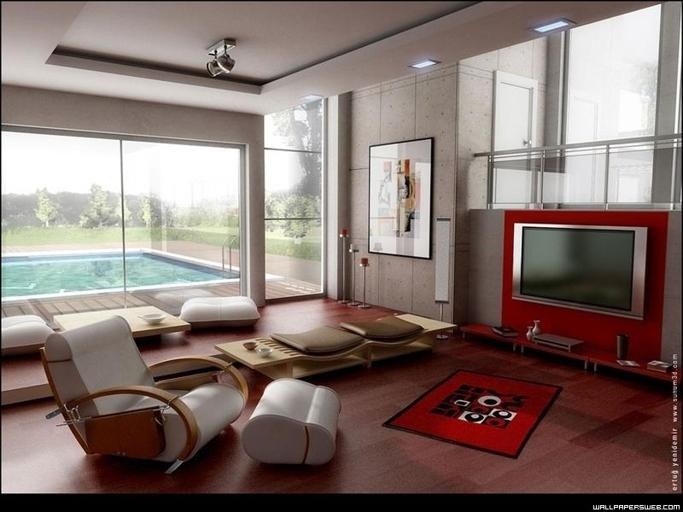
top-left (617, 335), bottom-right (628, 360)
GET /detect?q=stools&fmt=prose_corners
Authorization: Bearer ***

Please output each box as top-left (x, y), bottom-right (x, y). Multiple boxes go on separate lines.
top-left (0, 314), bottom-right (57, 356)
top-left (179, 296), bottom-right (261, 329)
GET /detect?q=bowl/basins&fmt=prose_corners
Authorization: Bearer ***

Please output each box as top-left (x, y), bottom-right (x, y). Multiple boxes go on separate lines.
top-left (138, 312), bottom-right (167, 324)
top-left (258, 346), bottom-right (273, 357)
top-left (242, 341), bottom-right (257, 350)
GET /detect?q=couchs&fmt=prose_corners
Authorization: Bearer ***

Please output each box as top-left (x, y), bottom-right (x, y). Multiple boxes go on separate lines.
top-left (39, 315), bottom-right (249, 475)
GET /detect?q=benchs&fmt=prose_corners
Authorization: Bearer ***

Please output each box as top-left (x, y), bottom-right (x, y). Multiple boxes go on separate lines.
top-left (214, 313), bottom-right (458, 381)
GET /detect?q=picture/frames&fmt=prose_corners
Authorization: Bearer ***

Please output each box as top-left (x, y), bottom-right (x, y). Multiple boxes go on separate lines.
top-left (368, 137), bottom-right (434, 260)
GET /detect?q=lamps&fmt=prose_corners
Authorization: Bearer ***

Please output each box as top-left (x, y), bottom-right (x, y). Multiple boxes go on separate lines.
top-left (206, 49), bottom-right (224, 78)
top-left (217, 46), bottom-right (235, 72)
top-left (346, 244), bottom-right (361, 306)
top-left (337, 228), bottom-right (350, 304)
top-left (359, 258), bottom-right (372, 309)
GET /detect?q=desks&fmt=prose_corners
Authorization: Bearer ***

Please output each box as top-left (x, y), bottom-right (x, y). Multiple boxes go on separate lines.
top-left (460, 322), bottom-right (683, 390)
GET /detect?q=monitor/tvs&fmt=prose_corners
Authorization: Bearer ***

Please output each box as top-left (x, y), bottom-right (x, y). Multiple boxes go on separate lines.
top-left (511, 221), bottom-right (649, 321)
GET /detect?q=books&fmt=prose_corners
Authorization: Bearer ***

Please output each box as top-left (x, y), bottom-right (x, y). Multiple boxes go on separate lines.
top-left (616, 359), bottom-right (640, 367)
top-left (646, 360), bottom-right (673, 373)
top-left (492, 326), bottom-right (519, 337)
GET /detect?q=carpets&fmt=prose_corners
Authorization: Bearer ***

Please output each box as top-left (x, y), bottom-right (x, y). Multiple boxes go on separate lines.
top-left (381, 368), bottom-right (564, 460)
